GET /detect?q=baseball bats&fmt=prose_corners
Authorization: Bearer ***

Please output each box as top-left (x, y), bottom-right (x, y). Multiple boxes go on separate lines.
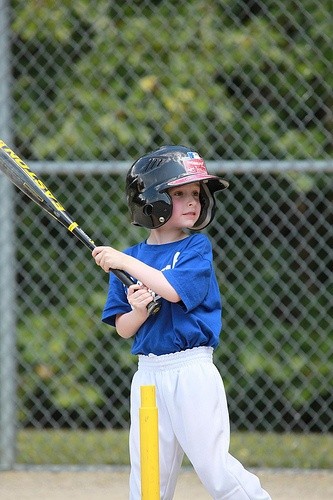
top-left (0, 138), bottom-right (156, 311)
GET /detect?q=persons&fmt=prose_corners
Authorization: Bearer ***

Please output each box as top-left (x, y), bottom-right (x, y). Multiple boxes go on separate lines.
top-left (91, 145), bottom-right (272, 500)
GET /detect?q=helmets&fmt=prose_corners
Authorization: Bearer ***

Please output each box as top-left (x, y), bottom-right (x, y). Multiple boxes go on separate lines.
top-left (126, 145), bottom-right (231, 231)
